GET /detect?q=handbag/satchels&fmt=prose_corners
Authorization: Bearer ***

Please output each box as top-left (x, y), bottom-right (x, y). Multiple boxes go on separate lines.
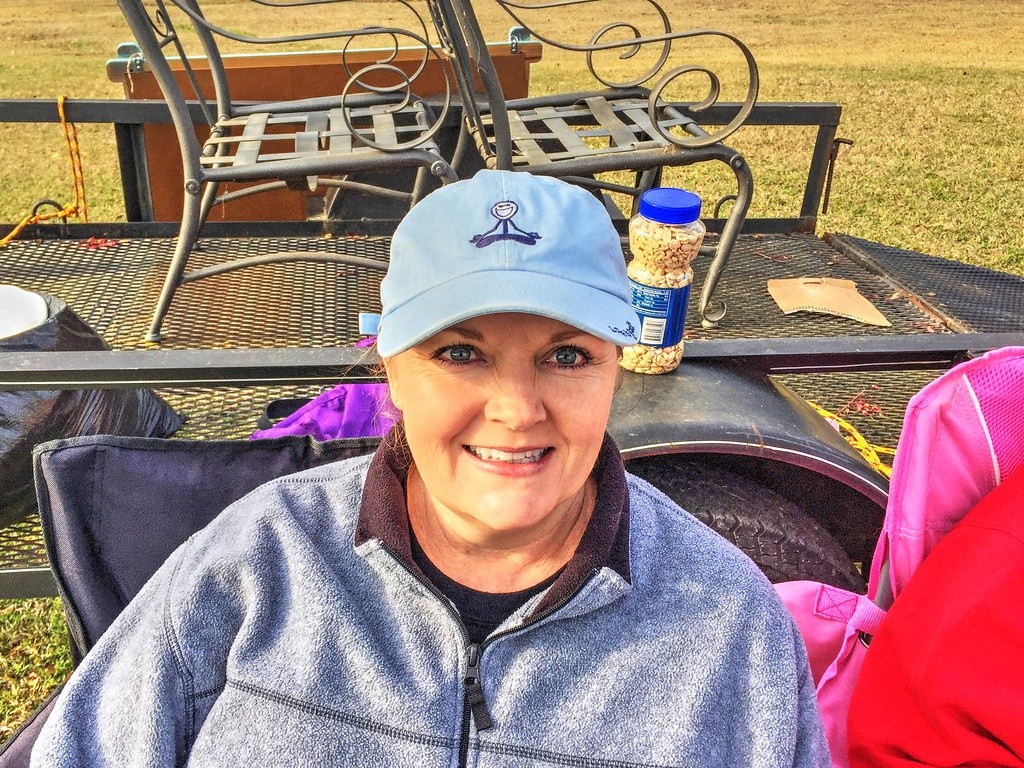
top-left (249, 335), bottom-right (400, 440)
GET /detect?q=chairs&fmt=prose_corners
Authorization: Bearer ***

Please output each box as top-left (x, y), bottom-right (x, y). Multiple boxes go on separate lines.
top-left (425, 0), bottom-right (759, 329)
top-left (32, 437), bottom-right (384, 670)
top-left (116, 0), bottom-right (457, 341)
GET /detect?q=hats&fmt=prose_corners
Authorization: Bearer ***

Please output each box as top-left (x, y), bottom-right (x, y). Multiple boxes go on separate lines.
top-left (358, 168), bottom-right (641, 359)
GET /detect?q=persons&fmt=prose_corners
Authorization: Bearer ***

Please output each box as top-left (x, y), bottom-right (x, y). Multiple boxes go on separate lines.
top-left (28, 169), bottom-right (834, 768)
top-left (846, 467), bottom-right (1024, 767)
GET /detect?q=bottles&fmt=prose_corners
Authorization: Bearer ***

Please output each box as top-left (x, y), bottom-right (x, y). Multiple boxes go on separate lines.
top-left (615, 187), bottom-right (707, 374)
